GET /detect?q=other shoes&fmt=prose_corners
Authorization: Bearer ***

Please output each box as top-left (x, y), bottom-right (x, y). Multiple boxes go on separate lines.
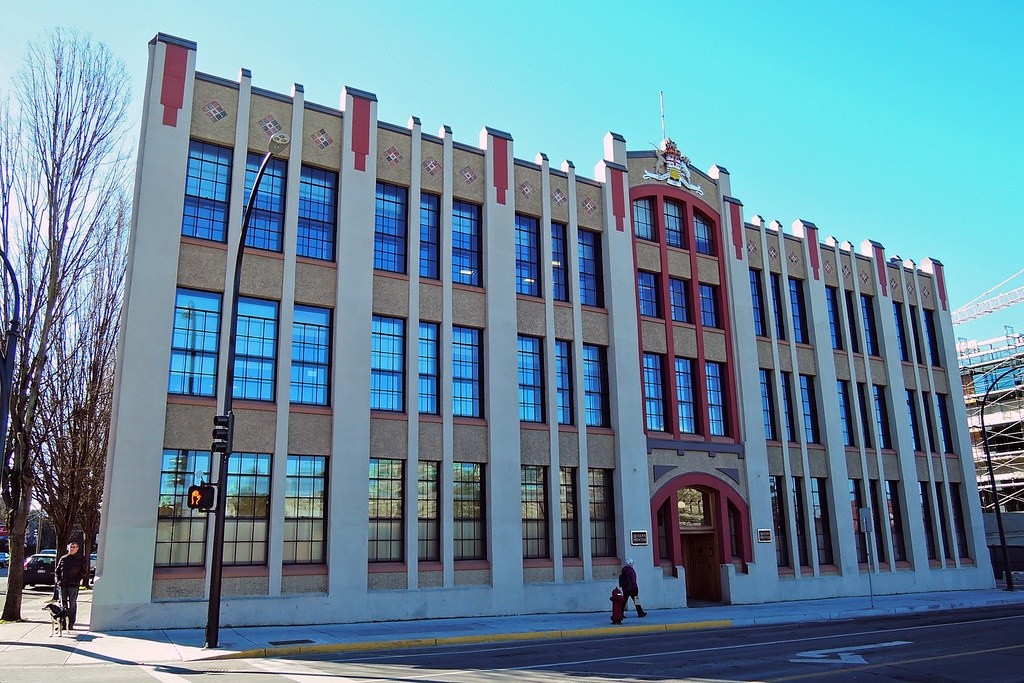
top-left (55, 621), bottom-right (66, 630)
top-left (68, 620), bottom-right (75, 629)
top-left (638, 612), bottom-right (647, 617)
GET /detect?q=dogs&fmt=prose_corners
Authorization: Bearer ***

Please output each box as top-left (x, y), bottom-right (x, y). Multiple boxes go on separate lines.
top-left (42, 603), bottom-right (72, 637)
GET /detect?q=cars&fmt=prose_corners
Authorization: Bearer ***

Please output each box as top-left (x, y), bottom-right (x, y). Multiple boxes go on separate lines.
top-left (90, 553), bottom-right (97, 572)
top-left (22, 554), bottom-right (57, 589)
top-left (37, 549), bottom-right (58, 567)
top-left (0, 551), bottom-right (9, 568)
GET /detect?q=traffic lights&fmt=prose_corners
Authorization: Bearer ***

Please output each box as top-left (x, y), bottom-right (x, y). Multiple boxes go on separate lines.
top-left (210, 414), bottom-right (236, 456)
top-left (187, 485), bottom-right (215, 510)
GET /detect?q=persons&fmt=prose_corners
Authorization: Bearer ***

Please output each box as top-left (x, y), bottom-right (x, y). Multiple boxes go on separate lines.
top-left (55, 541), bottom-right (87, 630)
top-left (621, 556), bottom-right (647, 618)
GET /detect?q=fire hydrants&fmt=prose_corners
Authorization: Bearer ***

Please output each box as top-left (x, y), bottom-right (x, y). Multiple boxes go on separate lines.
top-left (610, 587), bottom-right (626, 626)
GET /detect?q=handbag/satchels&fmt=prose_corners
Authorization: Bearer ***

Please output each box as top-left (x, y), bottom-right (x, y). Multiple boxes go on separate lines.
top-left (634, 595), bottom-right (641, 605)
top-left (618, 575), bottom-right (628, 587)
